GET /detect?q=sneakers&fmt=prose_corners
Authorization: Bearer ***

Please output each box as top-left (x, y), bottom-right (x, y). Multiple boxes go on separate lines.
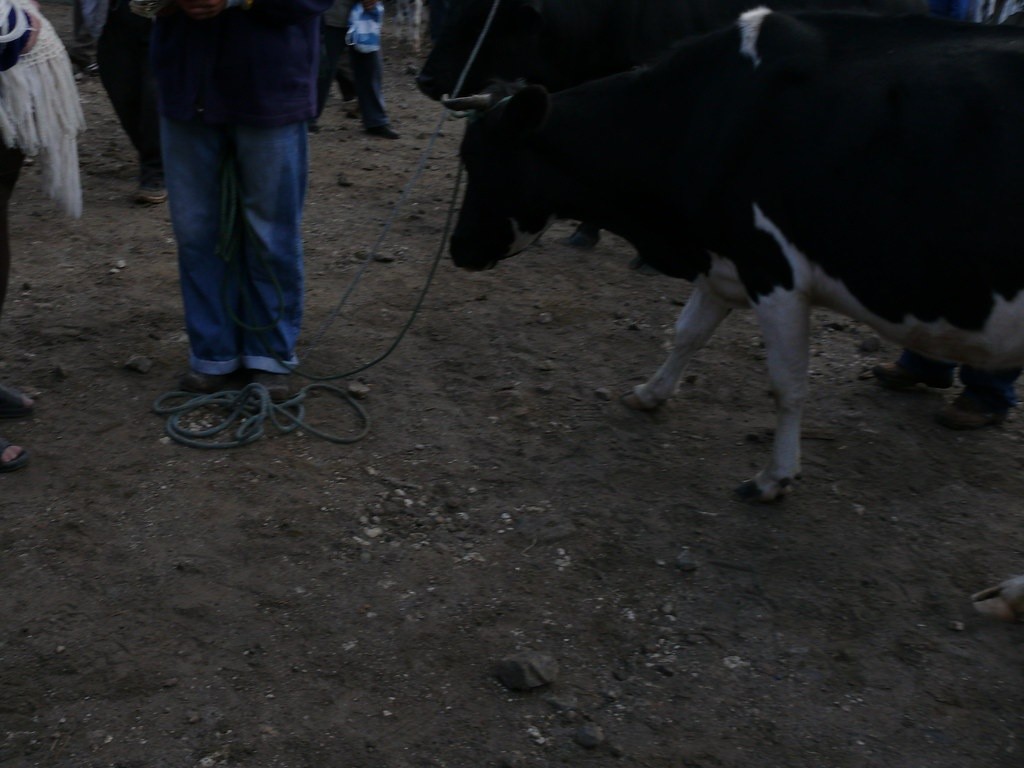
top-left (131, 158), bottom-right (180, 203)
top-left (935, 401), bottom-right (1008, 430)
top-left (873, 359), bottom-right (953, 404)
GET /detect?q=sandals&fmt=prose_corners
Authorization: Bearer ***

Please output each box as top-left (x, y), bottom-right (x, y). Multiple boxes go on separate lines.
top-left (0, 386), bottom-right (35, 418)
top-left (0, 437), bottom-right (30, 472)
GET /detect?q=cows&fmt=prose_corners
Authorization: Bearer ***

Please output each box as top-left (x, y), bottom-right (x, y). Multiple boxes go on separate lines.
top-left (415, 1), bottom-right (1023, 629)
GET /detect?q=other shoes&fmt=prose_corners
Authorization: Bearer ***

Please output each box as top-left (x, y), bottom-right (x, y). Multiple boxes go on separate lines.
top-left (179, 373), bottom-right (224, 393)
top-left (249, 371), bottom-right (289, 400)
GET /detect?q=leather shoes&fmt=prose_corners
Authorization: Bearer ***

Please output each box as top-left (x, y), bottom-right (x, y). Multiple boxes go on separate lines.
top-left (307, 122), bottom-right (319, 132)
top-left (363, 124), bottom-right (400, 139)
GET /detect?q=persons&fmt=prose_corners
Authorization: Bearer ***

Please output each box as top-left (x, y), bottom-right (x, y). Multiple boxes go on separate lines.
top-left (0, 0), bottom-right (42, 470)
top-left (72, 0), bottom-right (400, 404)
top-left (873, 0), bottom-right (1024, 428)
